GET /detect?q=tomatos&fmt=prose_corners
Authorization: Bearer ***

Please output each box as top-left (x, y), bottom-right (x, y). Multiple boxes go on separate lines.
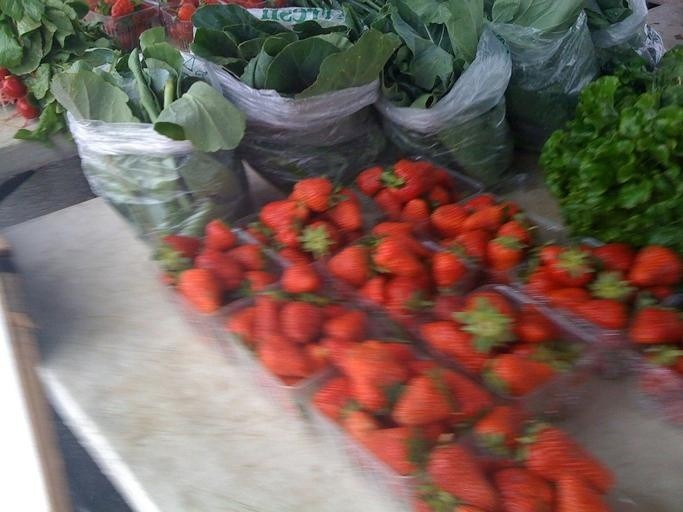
top-left (1, 67), bottom-right (40, 121)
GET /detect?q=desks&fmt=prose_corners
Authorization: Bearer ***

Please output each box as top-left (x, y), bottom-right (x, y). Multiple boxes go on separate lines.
top-left (0, 163), bottom-right (681, 512)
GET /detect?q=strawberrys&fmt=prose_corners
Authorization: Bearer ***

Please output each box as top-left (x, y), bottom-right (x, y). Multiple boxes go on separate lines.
top-left (84, 1), bottom-right (282, 47)
top-left (154, 158), bottom-right (683, 512)
